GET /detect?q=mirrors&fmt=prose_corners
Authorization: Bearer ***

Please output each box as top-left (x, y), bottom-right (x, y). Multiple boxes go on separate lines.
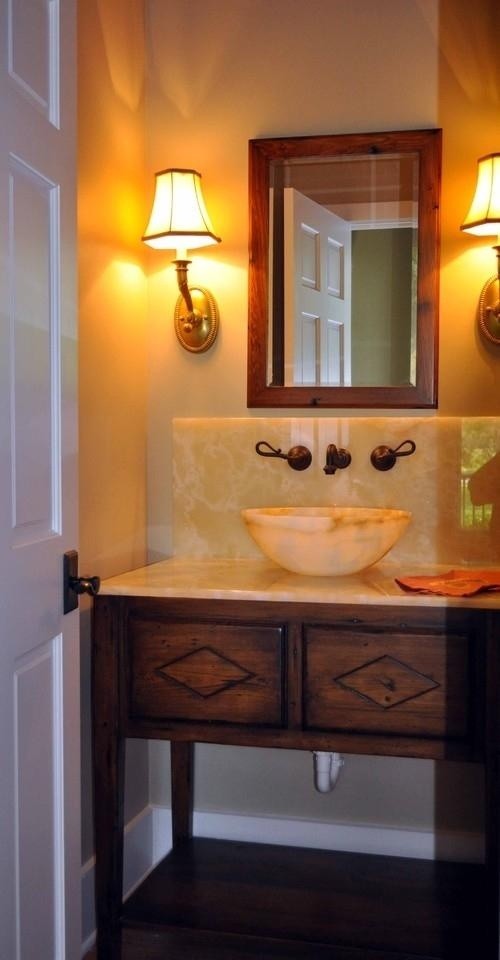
top-left (247, 128), bottom-right (443, 409)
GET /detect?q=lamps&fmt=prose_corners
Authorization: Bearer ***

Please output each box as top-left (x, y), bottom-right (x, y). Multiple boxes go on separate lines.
top-left (461, 153), bottom-right (500, 344)
top-left (142, 168), bottom-right (221, 354)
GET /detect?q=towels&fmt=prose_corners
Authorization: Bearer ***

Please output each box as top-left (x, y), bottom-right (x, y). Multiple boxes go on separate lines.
top-left (392, 570), bottom-right (499, 596)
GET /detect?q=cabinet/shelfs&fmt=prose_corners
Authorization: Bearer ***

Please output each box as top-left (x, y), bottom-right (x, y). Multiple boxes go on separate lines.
top-left (83, 594), bottom-right (500, 959)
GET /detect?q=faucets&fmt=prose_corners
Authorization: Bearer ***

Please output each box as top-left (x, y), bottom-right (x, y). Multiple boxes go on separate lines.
top-left (321, 443), bottom-right (351, 478)
